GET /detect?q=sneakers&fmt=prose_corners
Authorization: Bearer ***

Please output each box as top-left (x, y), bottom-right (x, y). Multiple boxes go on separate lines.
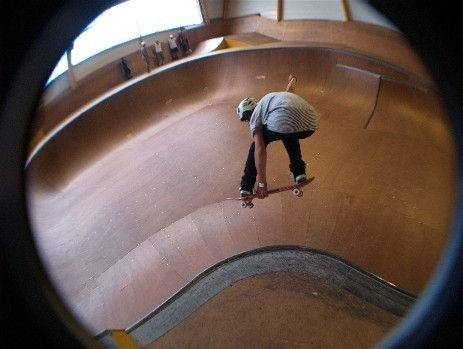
top-left (294, 173), bottom-right (307, 184)
top-left (239, 187), bottom-right (253, 197)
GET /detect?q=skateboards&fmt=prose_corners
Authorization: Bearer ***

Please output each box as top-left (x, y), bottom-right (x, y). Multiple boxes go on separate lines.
top-left (227, 177), bottom-right (315, 209)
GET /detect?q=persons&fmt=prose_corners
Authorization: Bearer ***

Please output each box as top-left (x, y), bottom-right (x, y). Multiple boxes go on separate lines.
top-left (236, 73), bottom-right (318, 199)
top-left (141, 41), bottom-right (151, 73)
top-left (154, 39), bottom-right (164, 66)
top-left (168, 34), bottom-right (178, 59)
top-left (179, 27), bottom-right (192, 54)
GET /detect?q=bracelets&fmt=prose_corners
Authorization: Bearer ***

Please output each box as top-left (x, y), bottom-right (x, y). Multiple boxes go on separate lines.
top-left (257, 182), bottom-right (267, 188)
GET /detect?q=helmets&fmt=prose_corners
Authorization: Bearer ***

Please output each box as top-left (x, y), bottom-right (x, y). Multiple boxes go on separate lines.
top-left (141, 41), bottom-right (145, 45)
top-left (169, 34), bottom-right (173, 38)
top-left (237, 97), bottom-right (257, 121)
